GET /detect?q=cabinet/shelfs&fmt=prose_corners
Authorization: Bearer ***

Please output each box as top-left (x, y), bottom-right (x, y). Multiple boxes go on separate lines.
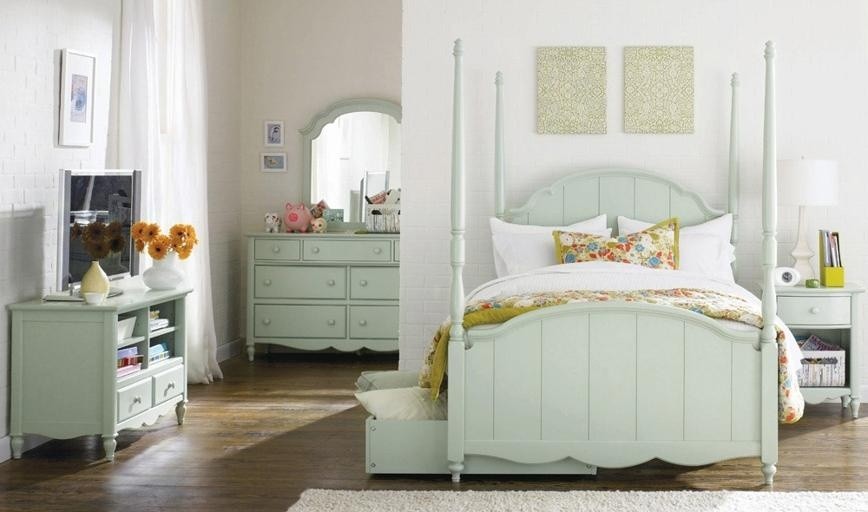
top-left (2, 281), bottom-right (199, 466)
top-left (243, 228), bottom-right (398, 361)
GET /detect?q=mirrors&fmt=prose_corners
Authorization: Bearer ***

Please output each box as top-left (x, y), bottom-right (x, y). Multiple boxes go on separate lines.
top-left (300, 95), bottom-right (401, 231)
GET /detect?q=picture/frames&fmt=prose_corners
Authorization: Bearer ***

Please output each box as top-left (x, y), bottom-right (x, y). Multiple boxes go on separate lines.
top-left (54, 46), bottom-right (98, 150)
top-left (262, 120), bottom-right (286, 147)
top-left (259, 151), bottom-right (288, 172)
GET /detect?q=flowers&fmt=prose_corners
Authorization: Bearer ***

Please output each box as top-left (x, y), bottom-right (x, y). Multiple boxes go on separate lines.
top-left (128, 218), bottom-right (202, 265)
top-left (68, 221), bottom-right (125, 262)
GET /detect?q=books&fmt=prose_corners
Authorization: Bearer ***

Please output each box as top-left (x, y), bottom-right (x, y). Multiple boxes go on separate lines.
top-left (822, 232), bottom-right (841, 267)
top-left (799, 333), bottom-right (837, 350)
top-left (116, 310), bottom-right (173, 380)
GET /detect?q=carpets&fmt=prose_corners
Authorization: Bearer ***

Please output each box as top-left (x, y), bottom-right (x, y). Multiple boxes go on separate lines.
top-left (280, 481), bottom-right (867, 511)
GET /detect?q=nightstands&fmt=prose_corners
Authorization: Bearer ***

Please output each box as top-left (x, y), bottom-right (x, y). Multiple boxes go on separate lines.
top-left (764, 280), bottom-right (867, 425)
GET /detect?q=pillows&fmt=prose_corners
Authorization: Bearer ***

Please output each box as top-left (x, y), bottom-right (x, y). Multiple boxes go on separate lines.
top-left (488, 215), bottom-right (608, 281)
top-left (551, 218), bottom-right (681, 269)
top-left (353, 368), bottom-right (427, 390)
top-left (351, 384), bottom-right (447, 423)
top-left (614, 214), bottom-right (739, 288)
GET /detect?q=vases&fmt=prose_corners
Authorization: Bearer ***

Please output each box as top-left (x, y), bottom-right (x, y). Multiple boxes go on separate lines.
top-left (80, 261), bottom-right (112, 302)
top-left (139, 254), bottom-right (182, 294)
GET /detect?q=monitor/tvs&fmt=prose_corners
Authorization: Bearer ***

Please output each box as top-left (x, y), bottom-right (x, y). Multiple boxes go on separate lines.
top-left (42, 167), bottom-right (142, 301)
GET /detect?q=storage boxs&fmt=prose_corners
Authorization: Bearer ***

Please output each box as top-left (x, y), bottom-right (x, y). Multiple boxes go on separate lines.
top-left (800, 348), bottom-right (846, 388)
top-left (363, 202), bottom-right (400, 232)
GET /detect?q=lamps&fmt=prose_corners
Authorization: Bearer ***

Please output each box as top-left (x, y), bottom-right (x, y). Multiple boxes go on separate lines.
top-left (764, 156), bottom-right (845, 286)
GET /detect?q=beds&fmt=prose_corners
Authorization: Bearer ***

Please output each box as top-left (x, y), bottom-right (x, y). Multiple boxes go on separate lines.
top-left (360, 33), bottom-right (788, 489)
